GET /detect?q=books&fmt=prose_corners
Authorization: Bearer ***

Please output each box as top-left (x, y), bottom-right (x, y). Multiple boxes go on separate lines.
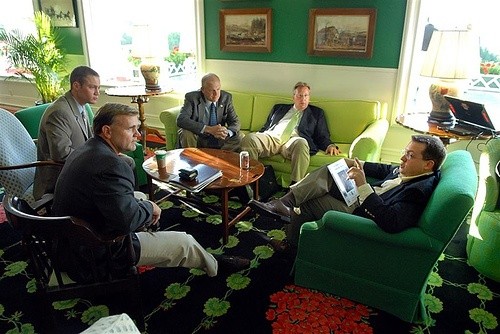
top-left (327, 158), bottom-right (359, 207)
top-left (169, 163), bottom-right (223, 193)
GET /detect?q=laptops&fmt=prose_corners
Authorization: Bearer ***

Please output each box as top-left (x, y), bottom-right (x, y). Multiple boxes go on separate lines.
top-left (441, 96), bottom-right (500, 135)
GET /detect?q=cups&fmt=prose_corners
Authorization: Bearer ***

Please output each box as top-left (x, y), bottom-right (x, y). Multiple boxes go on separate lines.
top-left (154, 150), bottom-right (167, 168)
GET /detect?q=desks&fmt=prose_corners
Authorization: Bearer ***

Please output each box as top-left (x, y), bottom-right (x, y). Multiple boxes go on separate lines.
top-left (105, 84), bottom-right (174, 152)
top-left (142, 148), bottom-right (264, 243)
top-left (396, 111), bottom-right (492, 145)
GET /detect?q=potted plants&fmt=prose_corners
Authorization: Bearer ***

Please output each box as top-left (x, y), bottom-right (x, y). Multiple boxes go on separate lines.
top-left (0, 10), bottom-right (73, 106)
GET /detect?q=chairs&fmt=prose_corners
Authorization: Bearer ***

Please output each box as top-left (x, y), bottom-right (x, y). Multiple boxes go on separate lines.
top-left (0, 108), bottom-right (54, 214)
top-left (294, 149), bottom-right (478, 323)
top-left (466, 136), bottom-right (500, 283)
top-left (13, 100), bottom-right (146, 186)
top-left (4, 195), bottom-right (137, 287)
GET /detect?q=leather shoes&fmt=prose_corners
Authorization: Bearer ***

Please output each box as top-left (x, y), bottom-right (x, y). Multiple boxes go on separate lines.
top-left (247, 196), bottom-right (291, 224)
top-left (268, 239), bottom-right (288, 256)
top-left (219, 256), bottom-right (249, 272)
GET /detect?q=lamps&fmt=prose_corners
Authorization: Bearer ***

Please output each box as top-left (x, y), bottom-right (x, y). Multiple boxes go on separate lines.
top-left (129, 31), bottom-right (167, 91)
top-left (419, 28), bottom-right (480, 125)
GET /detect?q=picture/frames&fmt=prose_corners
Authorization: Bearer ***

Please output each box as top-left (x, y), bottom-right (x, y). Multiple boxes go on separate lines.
top-left (218, 7), bottom-right (271, 52)
top-left (306, 7), bottom-right (375, 58)
top-left (37, 0), bottom-right (79, 28)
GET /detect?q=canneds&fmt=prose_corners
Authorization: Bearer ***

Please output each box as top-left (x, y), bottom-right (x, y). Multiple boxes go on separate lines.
top-left (240, 151), bottom-right (249, 169)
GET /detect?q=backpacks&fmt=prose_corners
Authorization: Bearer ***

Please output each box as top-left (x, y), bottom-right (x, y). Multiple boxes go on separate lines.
top-left (258, 165), bottom-right (278, 195)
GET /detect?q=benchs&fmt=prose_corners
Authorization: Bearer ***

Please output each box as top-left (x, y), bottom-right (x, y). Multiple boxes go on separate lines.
top-left (159, 88), bottom-right (390, 187)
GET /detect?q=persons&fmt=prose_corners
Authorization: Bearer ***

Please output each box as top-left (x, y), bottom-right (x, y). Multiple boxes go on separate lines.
top-left (32, 66), bottom-right (140, 202)
top-left (247, 135), bottom-right (447, 263)
top-left (176, 73), bottom-right (245, 152)
top-left (47, 103), bottom-right (250, 278)
top-left (240, 82), bottom-right (342, 198)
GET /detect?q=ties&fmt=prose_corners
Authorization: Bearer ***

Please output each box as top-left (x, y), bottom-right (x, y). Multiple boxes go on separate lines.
top-left (81, 110), bottom-right (88, 134)
top-left (281, 112), bottom-right (300, 146)
top-left (209, 103), bottom-right (216, 127)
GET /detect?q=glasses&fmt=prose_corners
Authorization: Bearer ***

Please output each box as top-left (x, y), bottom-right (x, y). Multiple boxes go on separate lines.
top-left (401, 149), bottom-right (422, 161)
